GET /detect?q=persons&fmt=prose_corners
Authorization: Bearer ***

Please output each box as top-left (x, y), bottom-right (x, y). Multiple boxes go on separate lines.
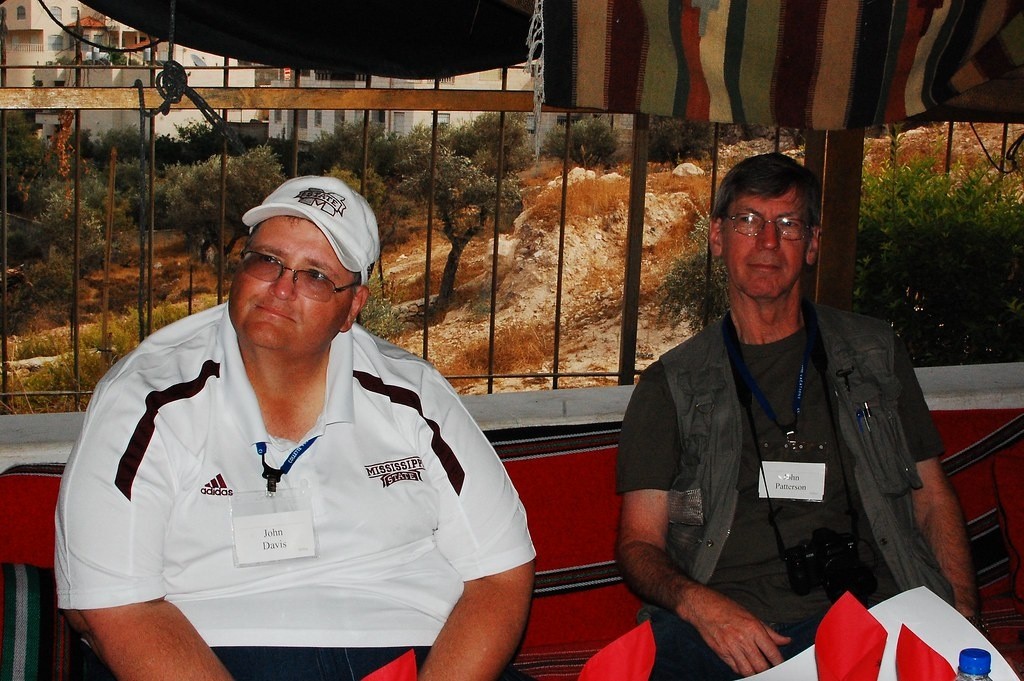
top-left (607, 151), bottom-right (975, 681)
top-left (54, 176), bottom-right (546, 681)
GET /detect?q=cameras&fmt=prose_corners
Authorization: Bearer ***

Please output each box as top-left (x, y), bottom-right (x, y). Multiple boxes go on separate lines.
top-left (786, 527), bottom-right (878, 606)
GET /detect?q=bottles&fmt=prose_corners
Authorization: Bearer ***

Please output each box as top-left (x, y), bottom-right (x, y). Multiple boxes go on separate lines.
top-left (953, 647), bottom-right (994, 681)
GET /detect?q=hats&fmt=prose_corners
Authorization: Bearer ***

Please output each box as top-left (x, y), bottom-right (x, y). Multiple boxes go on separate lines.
top-left (241, 175), bottom-right (381, 286)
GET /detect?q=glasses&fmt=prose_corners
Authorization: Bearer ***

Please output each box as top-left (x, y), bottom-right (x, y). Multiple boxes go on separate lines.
top-left (725, 211), bottom-right (811, 240)
top-left (240, 249), bottom-right (357, 302)
